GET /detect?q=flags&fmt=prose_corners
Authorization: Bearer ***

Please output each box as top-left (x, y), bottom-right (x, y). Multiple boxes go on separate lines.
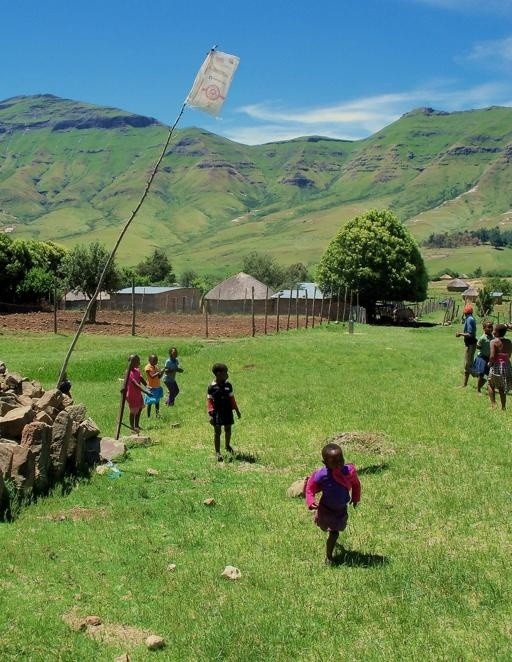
top-left (182, 49), bottom-right (240, 118)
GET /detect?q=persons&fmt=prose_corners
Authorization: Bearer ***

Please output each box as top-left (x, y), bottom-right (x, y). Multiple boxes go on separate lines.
top-left (486, 323), bottom-right (512, 410)
top-left (305, 443), bottom-right (363, 565)
top-left (144, 353), bottom-right (167, 418)
top-left (455, 304), bottom-right (485, 389)
top-left (162, 347), bottom-right (184, 407)
top-left (124, 354), bottom-right (155, 434)
top-left (207, 363), bottom-right (241, 461)
top-left (469, 319), bottom-right (495, 397)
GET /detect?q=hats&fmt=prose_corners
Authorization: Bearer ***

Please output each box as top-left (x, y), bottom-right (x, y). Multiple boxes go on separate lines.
top-left (464, 305), bottom-right (473, 313)
